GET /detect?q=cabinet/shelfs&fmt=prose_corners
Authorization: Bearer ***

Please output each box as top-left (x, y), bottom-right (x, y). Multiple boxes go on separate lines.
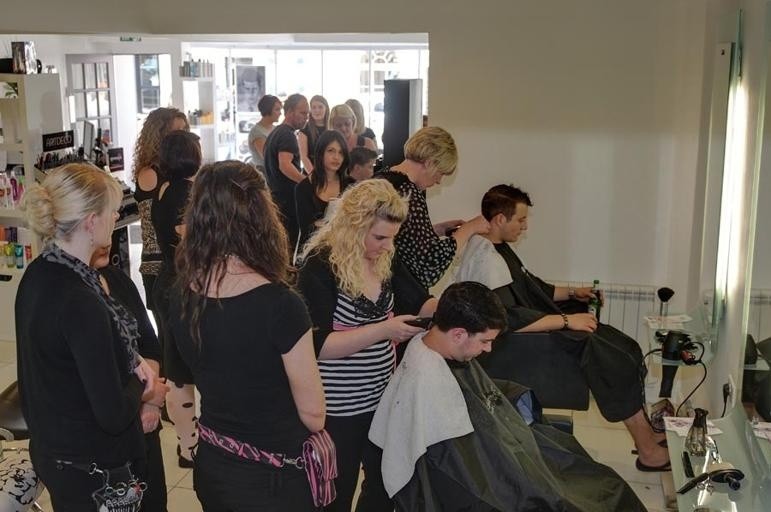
top-left (0, 74), bottom-right (70, 339)
top-left (181, 76), bottom-right (214, 129)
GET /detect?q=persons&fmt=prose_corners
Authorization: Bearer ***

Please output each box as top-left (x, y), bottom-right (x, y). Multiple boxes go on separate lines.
top-left (14, 162), bottom-right (169, 511)
top-left (367, 281), bottom-right (649, 512)
top-left (93, 242), bottom-right (170, 511)
top-left (448, 183), bottom-right (671, 473)
top-left (291, 178), bottom-right (439, 511)
top-left (247, 92), bottom-right (383, 265)
top-left (131, 106), bottom-right (191, 336)
top-left (165, 161), bottom-right (327, 511)
top-left (150, 129), bottom-right (203, 470)
top-left (373, 127), bottom-right (490, 288)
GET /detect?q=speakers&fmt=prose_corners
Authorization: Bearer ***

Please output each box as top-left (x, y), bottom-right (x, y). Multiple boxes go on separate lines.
top-left (384, 79), bottom-right (422, 170)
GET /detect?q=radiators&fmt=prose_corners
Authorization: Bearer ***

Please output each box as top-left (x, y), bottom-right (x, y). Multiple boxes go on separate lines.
top-left (547, 281), bottom-right (656, 366)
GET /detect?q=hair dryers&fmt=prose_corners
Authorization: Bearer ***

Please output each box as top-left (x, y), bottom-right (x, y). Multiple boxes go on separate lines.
top-left (658, 329), bottom-right (696, 398)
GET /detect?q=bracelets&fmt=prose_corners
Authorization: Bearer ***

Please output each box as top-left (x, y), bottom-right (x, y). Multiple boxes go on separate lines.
top-left (561, 313), bottom-right (568, 331)
top-left (569, 287), bottom-right (576, 300)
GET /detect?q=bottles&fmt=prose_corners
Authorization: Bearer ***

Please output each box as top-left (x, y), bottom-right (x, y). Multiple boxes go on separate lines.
top-left (190, 109), bottom-right (212, 125)
top-left (189, 59), bottom-right (210, 78)
top-left (684, 407), bottom-right (709, 456)
top-left (586, 279), bottom-right (602, 323)
top-left (0, 171), bottom-right (23, 209)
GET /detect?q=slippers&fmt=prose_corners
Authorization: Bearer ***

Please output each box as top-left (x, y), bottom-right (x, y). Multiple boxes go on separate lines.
top-left (631, 440), bottom-right (672, 472)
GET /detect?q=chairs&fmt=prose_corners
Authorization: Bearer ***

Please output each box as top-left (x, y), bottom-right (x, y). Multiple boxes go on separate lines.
top-left (483, 333), bottom-right (590, 410)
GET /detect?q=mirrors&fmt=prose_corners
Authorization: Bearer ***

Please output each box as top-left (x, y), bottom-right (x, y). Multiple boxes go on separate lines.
top-left (742, 85), bottom-right (771, 476)
top-left (700, 41), bottom-right (734, 326)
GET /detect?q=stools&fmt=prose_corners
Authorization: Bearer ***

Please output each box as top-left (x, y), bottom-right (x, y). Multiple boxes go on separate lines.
top-left (0, 427), bottom-right (45, 512)
top-left (1, 380), bottom-right (33, 439)
